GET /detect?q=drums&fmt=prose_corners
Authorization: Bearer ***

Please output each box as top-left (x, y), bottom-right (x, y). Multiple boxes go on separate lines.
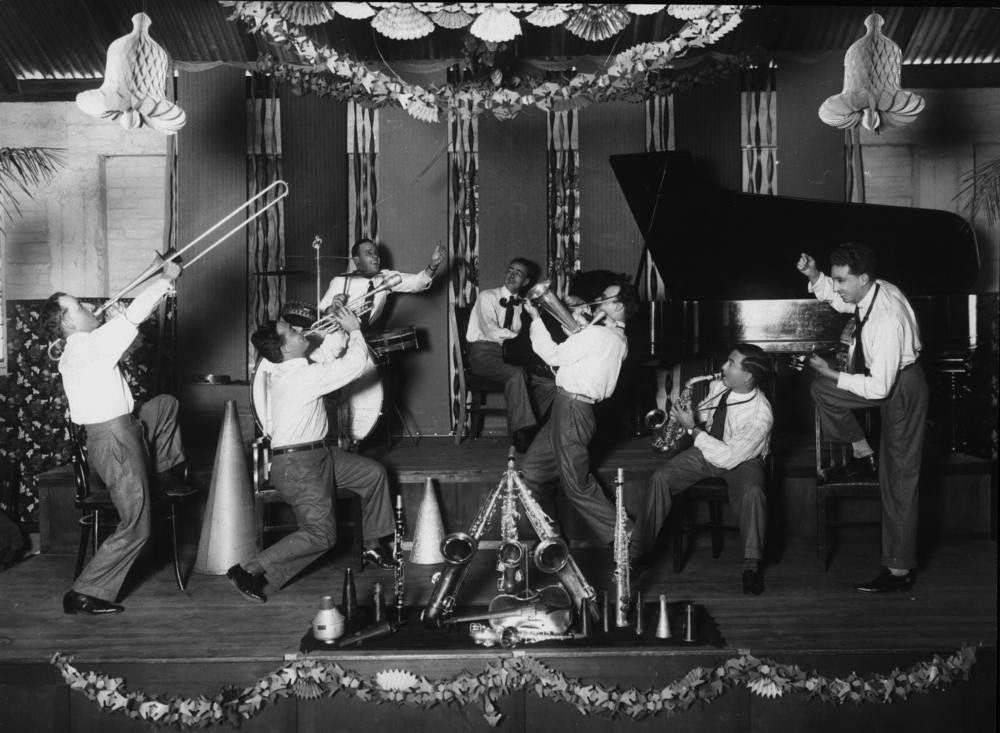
top-left (361, 325), bottom-right (422, 356)
top-left (281, 300), bottom-right (318, 326)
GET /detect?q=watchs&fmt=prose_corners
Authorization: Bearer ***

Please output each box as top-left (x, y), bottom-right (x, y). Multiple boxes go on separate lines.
top-left (686, 424), bottom-right (697, 437)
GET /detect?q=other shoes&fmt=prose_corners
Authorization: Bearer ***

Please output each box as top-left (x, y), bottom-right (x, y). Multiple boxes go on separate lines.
top-left (744, 560), bottom-right (763, 591)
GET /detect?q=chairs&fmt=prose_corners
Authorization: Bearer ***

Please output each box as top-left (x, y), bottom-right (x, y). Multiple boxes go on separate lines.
top-left (67, 418), bottom-right (189, 595)
top-left (815, 398), bottom-right (884, 571)
top-left (670, 372), bottom-right (785, 572)
top-left (450, 302), bottom-right (508, 443)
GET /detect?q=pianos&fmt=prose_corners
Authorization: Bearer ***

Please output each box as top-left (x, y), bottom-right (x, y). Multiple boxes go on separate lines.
top-left (609, 149), bottom-right (990, 467)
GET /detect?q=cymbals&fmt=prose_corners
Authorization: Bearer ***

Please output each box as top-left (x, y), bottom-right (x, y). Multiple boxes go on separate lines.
top-left (252, 269), bottom-right (310, 275)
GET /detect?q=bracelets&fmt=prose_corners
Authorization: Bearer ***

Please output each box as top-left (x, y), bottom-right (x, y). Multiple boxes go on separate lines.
top-left (428, 264), bottom-right (439, 272)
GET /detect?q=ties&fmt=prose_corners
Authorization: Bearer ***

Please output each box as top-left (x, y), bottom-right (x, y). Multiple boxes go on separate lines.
top-left (854, 307), bottom-right (865, 372)
top-left (710, 390), bottom-right (732, 440)
top-left (360, 281), bottom-right (375, 329)
top-left (504, 296), bottom-right (514, 329)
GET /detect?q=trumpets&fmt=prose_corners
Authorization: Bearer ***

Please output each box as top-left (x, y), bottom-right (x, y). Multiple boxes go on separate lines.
top-left (300, 274), bottom-right (393, 342)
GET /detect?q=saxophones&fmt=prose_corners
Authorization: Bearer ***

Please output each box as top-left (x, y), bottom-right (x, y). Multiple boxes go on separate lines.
top-left (642, 371), bottom-right (726, 453)
top-left (612, 466), bottom-right (636, 626)
top-left (420, 468), bottom-right (603, 627)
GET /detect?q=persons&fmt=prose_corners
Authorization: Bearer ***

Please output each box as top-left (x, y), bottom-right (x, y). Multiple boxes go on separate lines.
top-left (797, 240), bottom-right (929, 594)
top-left (465, 256), bottom-right (571, 452)
top-left (319, 239), bottom-right (446, 332)
top-left (621, 346), bottom-right (774, 596)
top-left (517, 280), bottom-right (640, 557)
top-left (35, 263), bottom-right (185, 619)
top-left (226, 305), bottom-right (411, 604)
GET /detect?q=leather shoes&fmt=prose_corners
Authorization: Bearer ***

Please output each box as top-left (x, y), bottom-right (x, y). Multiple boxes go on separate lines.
top-left (362, 547), bottom-right (398, 569)
top-left (63, 592), bottom-right (123, 614)
top-left (855, 568), bottom-right (914, 592)
top-left (226, 563), bottom-right (265, 602)
top-left (832, 453), bottom-right (879, 477)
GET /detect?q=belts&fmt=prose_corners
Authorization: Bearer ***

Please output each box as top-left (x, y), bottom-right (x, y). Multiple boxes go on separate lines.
top-left (273, 443), bottom-right (324, 456)
top-left (556, 388), bottom-right (595, 404)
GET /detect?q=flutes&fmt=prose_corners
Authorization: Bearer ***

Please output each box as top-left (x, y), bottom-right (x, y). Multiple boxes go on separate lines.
top-left (392, 494), bottom-right (405, 627)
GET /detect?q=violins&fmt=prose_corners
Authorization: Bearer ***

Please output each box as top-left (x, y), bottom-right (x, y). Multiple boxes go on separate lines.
top-left (499, 298), bottom-right (593, 327)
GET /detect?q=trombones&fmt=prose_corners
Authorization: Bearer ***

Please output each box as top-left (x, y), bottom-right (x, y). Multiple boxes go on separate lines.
top-left (46, 178), bottom-right (288, 360)
